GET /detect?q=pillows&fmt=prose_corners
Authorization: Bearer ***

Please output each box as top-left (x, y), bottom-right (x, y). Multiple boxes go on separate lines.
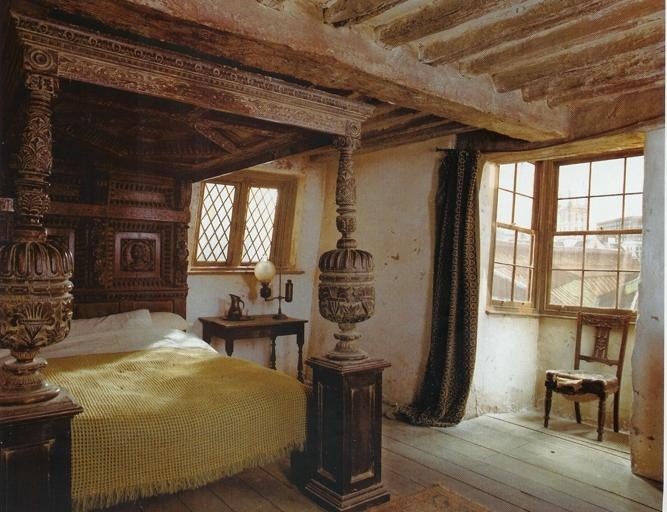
top-left (148, 312), bottom-right (188, 331)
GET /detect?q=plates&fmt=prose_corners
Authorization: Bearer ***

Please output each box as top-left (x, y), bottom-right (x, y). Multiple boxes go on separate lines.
top-left (221, 316), bottom-right (254, 321)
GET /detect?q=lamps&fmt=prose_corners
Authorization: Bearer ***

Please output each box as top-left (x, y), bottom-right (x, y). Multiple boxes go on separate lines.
top-left (254, 260), bottom-right (295, 320)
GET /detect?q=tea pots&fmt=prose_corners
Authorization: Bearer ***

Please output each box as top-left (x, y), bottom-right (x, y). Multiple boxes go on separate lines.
top-left (227, 293), bottom-right (246, 319)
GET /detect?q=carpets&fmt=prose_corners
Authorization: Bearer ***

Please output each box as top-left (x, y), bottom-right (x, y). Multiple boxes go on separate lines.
top-left (361, 483), bottom-right (494, 511)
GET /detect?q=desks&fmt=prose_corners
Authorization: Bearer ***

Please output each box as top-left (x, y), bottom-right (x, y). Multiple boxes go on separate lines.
top-left (199, 315), bottom-right (310, 384)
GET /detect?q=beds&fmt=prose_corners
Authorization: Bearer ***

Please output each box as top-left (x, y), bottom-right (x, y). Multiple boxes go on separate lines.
top-left (0, 308), bottom-right (313, 512)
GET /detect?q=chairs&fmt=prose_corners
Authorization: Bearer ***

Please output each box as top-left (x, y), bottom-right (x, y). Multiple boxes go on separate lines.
top-left (544, 310), bottom-right (634, 440)
